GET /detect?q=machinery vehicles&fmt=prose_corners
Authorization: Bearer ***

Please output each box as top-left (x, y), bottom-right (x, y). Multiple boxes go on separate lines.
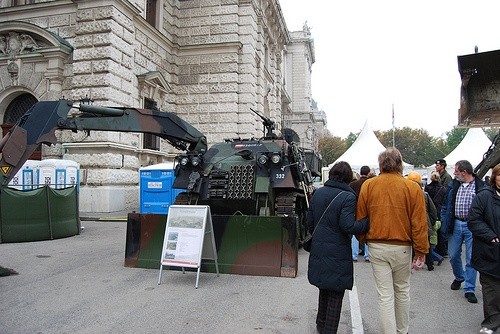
top-left (0, 96), bottom-right (322, 279)
top-left (456, 45), bottom-right (500, 181)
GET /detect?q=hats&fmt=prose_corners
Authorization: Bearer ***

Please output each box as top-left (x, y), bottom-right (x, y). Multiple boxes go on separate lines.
top-left (409, 171), bottom-right (422, 182)
top-left (435, 160), bottom-right (447, 164)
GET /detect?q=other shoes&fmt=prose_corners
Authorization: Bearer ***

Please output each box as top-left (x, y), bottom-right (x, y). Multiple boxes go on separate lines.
top-left (464, 293), bottom-right (478, 303)
top-left (478, 328), bottom-right (495, 334)
top-left (450, 279), bottom-right (465, 290)
top-left (365, 260), bottom-right (370, 263)
top-left (428, 266), bottom-right (435, 271)
top-left (437, 258), bottom-right (444, 265)
top-left (353, 260), bottom-right (357, 263)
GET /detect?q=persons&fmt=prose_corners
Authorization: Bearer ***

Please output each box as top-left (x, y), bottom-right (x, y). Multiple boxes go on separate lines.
top-left (307, 161), bottom-right (368, 334)
top-left (349, 160), bottom-right (453, 271)
top-left (484, 176), bottom-right (490, 183)
top-left (467, 165), bottom-right (500, 334)
top-left (356, 149), bottom-right (430, 334)
top-left (441, 160), bottom-right (485, 303)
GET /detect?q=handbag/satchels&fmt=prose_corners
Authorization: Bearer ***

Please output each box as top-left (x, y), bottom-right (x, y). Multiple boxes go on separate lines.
top-left (301, 234), bottom-right (312, 252)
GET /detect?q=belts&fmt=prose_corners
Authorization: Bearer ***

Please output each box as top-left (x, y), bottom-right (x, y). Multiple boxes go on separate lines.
top-left (456, 217), bottom-right (468, 222)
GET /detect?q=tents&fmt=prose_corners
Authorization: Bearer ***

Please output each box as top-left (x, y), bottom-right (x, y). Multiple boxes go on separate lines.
top-left (329, 120), bottom-right (414, 175)
top-left (427, 128), bottom-right (495, 169)
